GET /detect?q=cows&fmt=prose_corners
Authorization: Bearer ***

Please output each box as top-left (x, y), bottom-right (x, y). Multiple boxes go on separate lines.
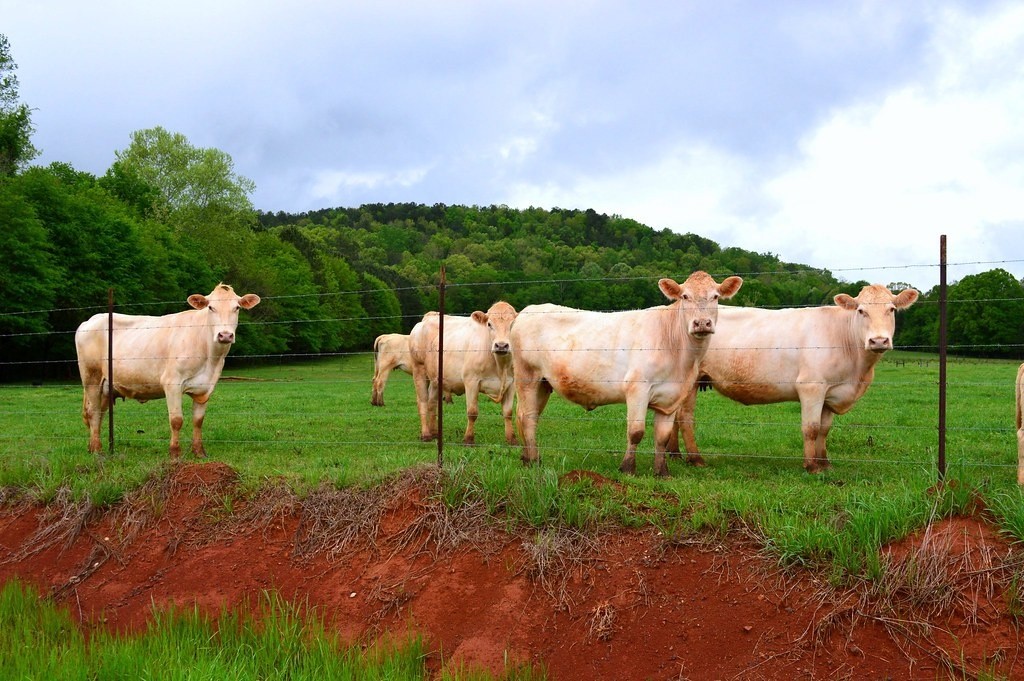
top-left (368, 271), bottom-right (919, 477)
top-left (1015, 364), bottom-right (1024, 486)
top-left (74, 284), bottom-right (260, 462)
